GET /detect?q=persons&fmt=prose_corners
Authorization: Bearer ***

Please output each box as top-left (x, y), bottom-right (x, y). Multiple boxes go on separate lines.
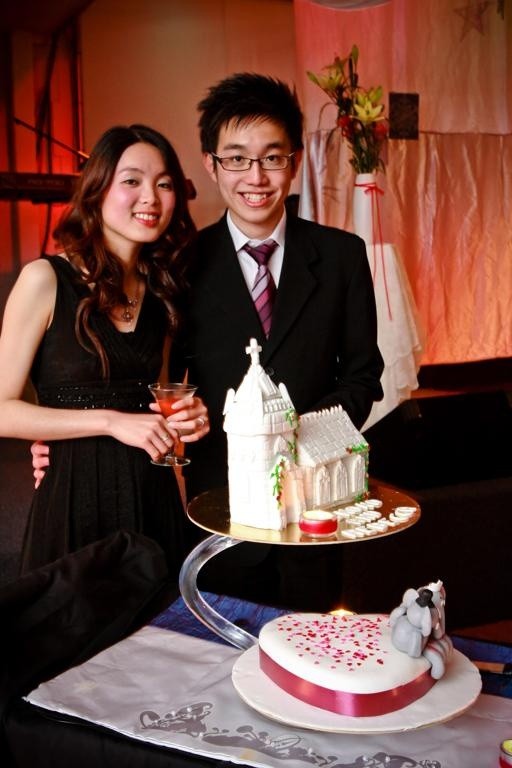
top-left (30, 73), bottom-right (384, 490)
top-left (1, 124), bottom-right (210, 573)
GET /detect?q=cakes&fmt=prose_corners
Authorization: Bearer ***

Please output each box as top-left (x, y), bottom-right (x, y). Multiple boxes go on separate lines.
top-left (258, 579), bottom-right (453, 719)
top-left (220, 336), bottom-right (418, 539)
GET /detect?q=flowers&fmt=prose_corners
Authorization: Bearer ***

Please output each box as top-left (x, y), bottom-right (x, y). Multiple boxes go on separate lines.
top-left (305, 41), bottom-right (392, 177)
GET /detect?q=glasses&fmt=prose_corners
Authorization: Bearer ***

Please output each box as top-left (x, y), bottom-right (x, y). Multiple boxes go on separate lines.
top-left (211, 150), bottom-right (296, 170)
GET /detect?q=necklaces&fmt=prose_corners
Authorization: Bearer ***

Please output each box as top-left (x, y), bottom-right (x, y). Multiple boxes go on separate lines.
top-left (120, 279), bottom-right (141, 323)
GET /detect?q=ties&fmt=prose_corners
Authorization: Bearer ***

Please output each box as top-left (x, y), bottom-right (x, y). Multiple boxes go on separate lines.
top-left (243, 241), bottom-right (275, 337)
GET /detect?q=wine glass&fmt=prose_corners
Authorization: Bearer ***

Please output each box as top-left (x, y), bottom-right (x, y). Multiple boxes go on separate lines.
top-left (146, 383), bottom-right (199, 470)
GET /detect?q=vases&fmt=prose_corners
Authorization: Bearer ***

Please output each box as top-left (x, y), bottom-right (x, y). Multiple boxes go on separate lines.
top-left (352, 174), bottom-right (379, 246)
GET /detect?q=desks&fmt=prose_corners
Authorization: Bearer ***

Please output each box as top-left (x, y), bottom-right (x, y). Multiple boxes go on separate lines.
top-left (2, 588), bottom-right (511, 768)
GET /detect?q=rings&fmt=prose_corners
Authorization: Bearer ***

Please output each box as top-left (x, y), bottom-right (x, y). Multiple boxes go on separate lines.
top-left (198, 417), bottom-right (205, 427)
top-left (162, 433), bottom-right (169, 442)
